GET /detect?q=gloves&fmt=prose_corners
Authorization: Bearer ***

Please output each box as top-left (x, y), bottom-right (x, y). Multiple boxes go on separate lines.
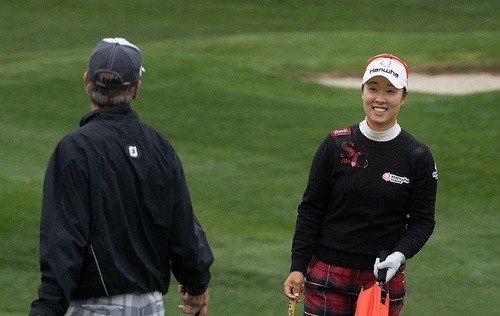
top-left (374, 252), bottom-right (406, 283)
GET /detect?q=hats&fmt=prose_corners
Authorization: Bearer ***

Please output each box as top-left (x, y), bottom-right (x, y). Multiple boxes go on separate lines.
top-left (88, 37), bottom-right (145, 89)
top-left (362, 53), bottom-right (408, 93)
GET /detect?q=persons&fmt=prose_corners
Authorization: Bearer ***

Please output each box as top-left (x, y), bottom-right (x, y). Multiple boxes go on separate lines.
top-left (29, 37), bottom-right (214, 316)
top-left (283, 54), bottom-right (438, 316)
